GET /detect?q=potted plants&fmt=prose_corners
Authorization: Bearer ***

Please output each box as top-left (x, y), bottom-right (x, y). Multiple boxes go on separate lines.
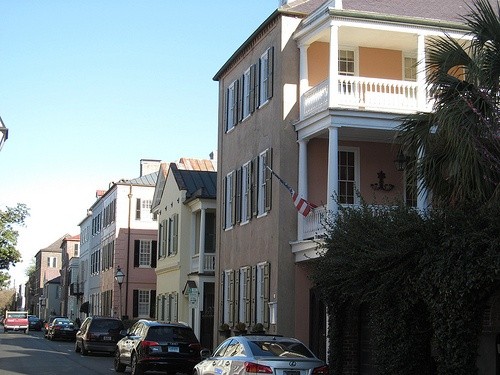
top-left (250, 323), bottom-right (266, 335)
top-left (233, 322), bottom-right (247, 336)
top-left (218, 323), bottom-right (231, 336)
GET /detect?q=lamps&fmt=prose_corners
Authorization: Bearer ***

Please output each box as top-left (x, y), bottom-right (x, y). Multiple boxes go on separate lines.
top-left (394, 147), bottom-right (410, 171)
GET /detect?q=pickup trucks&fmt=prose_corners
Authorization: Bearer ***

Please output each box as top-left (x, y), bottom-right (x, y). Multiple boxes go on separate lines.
top-left (3, 310), bottom-right (42, 334)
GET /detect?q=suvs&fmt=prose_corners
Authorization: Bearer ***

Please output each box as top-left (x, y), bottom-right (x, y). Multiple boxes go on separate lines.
top-left (114, 320), bottom-right (201, 375)
top-left (74, 316), bottom-right (126, 357)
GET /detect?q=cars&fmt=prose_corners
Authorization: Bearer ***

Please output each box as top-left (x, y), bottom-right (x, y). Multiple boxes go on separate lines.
top-left (192, 336), bottom-right (329, 375)
top-left (43, 316), bottom-right (80, 342)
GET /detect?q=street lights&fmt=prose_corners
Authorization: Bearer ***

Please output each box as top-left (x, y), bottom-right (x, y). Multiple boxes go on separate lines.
top-left (114, 265), bottom-right (125, 320)
